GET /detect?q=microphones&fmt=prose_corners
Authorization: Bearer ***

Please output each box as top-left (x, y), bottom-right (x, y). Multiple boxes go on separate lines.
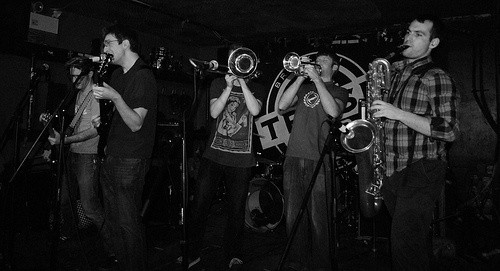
top-left (190, 57), bottom-right (219, 70)
top-left (31, 64), bottom-right (49, 70)
top-left (325, 118), bottom-right (355, 139)
top-left (80, 54), bottom-right (107, 63)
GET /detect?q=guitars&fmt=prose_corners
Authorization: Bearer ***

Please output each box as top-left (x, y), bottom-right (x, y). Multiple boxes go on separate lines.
top-left (39, 113), bottom-right (55, 137)
top-left (97, 54), bottom-right (113, 159)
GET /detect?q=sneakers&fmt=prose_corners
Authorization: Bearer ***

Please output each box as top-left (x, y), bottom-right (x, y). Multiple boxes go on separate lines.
top-left (228, 253), bottom-right (245, 269)
top-left (172, 255), bottom-right (201, 271)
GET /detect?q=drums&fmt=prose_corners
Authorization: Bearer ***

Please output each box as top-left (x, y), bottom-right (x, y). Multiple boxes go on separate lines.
top-left (244, 178), bottom-right (284, 232)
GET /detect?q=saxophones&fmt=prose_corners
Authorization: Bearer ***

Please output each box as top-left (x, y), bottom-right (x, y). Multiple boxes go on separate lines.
top-left (340, 45), bottom-right (409, 219)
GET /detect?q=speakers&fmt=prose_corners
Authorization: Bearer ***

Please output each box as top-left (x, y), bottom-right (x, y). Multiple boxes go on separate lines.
top-left (153, 123), bottom-right (185, 219)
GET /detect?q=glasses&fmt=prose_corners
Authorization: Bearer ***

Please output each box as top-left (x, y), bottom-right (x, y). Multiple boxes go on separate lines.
top-left (103, 39), bottom-right (124, 47)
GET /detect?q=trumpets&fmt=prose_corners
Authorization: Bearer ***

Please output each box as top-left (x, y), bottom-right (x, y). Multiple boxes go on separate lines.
top-left (283, 52), bottom-right (322, 79)
top-left (227, 47), bottom-right (261, 83)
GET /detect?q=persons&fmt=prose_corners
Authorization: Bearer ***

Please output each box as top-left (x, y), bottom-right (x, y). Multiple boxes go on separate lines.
top-left (187, 43), bottom-right (266, 270)
top-left (40, 56), bottom-right (121, 264)
top-left (278, 50), bottom-right (348, 271)
top-left (92, 25), bottom-right (158, 270)
top-left (370, 14), bottom-right (459, 270)
